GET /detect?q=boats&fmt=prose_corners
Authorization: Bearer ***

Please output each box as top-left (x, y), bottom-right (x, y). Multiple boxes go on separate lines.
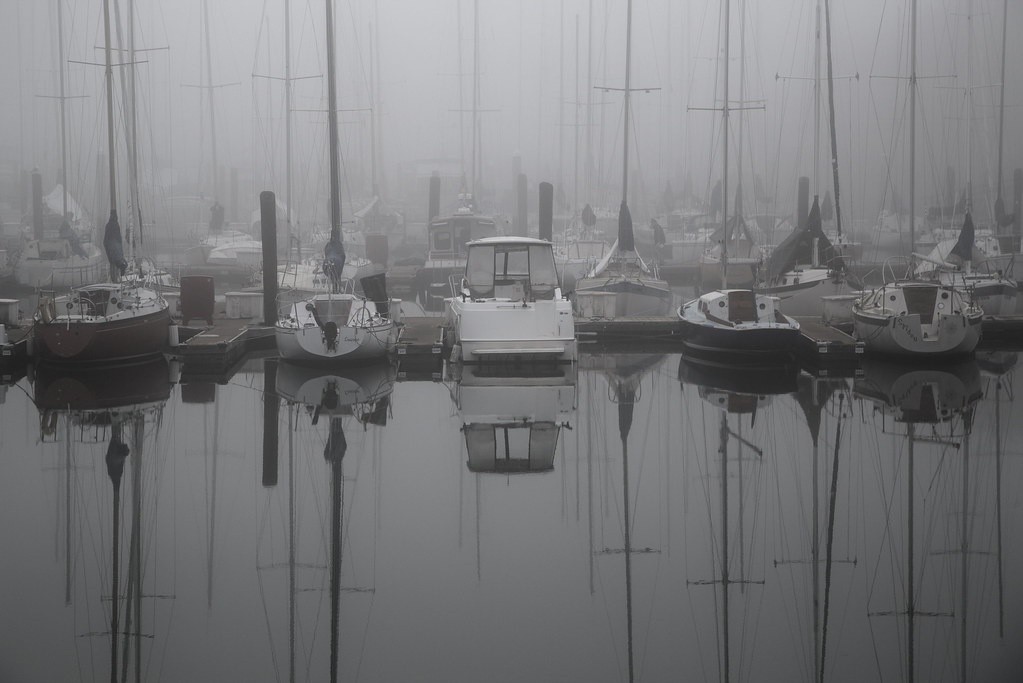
top-left (446, 236), bottom-right (579, 361)
top-left (441, 362), bottom-right (578, 476)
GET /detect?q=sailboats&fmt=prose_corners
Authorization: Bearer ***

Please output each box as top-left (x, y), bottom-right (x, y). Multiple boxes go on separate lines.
top-left (0, 370), bottom-right (1023, 683)
top-left (0, 0), bottom-right (1023, 371)
